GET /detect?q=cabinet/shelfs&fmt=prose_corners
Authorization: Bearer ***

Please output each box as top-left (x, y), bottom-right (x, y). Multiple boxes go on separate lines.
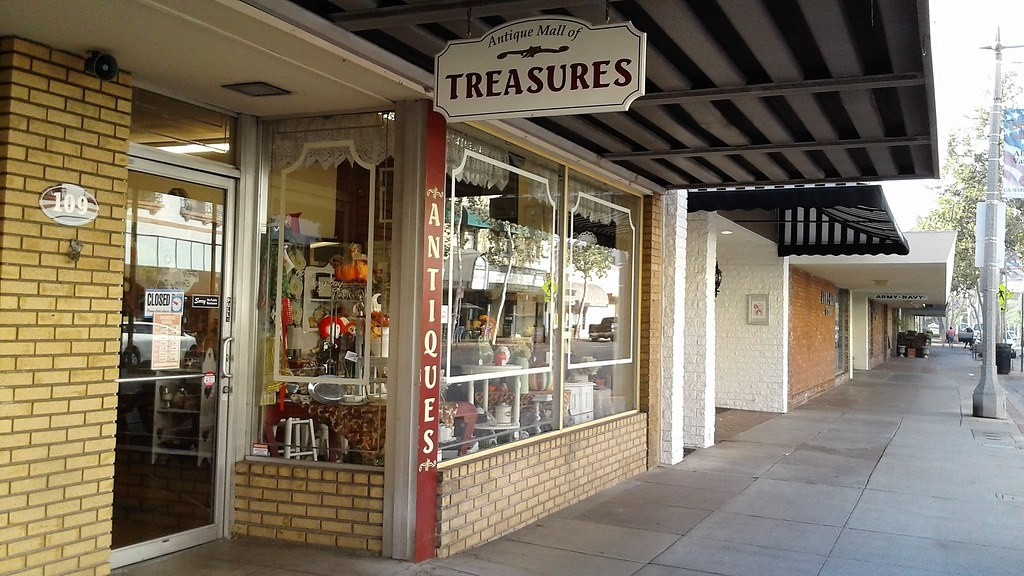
top-left (566, 381), bottom-right (595, 426)
top-left (450, 363), bottom-right (521, 442)
top-left (303, 263), bottom-right (342, 333)
top-left (116, 366), bottom-right (218, 467)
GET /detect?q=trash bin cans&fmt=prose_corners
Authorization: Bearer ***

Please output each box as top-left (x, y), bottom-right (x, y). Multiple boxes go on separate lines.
top-left (996, 343), bottom-right (1013, 375)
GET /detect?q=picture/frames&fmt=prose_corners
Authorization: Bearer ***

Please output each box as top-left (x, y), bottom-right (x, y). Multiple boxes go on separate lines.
top-left (746, 294), bottom-right (771, 326)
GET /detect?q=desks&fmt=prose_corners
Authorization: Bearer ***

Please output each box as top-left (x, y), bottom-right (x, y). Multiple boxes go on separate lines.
top-left (473, 390), bottom-right (570, 437)
top-left (276, 398), bottom-right (386, 464)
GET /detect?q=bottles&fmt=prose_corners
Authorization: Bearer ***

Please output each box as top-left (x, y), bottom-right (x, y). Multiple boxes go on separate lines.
top-left (320, 350), bottom-right (337, 375)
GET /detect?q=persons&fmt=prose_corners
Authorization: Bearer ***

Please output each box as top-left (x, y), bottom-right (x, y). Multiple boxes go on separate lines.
top-left (964, 327), bottom-right (974, 348)
top-left (947, 326), bottom-right (955, 348)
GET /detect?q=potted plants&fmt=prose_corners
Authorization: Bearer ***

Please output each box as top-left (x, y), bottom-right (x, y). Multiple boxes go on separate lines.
top-left (894, 314), bottom-right (910, 358)
top-left (920, 331), bottom-right (934, 359)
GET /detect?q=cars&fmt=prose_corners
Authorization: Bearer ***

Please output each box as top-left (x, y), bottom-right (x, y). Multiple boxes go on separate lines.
top-left (122, 322), bottom-right (198, 368)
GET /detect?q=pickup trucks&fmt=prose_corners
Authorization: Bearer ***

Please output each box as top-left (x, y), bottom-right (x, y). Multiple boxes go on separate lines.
top-left (588, 316), bottom-right (617, 341)
